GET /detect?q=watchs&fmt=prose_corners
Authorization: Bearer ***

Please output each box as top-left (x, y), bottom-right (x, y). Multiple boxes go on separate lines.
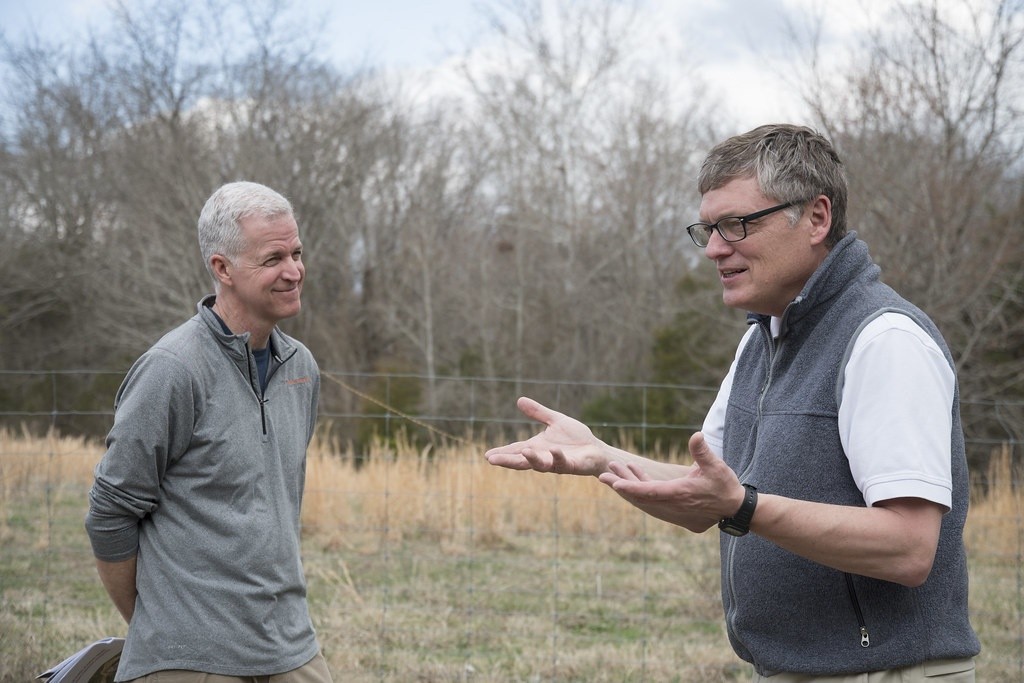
top-left (718, 484), bottom-right (758, 537)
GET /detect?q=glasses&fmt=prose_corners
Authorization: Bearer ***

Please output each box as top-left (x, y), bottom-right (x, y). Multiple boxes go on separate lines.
top-left (687, 197), bottom-right (813, 247)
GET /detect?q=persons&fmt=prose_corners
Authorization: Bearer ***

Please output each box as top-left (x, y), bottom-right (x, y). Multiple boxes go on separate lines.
top-left (85, 182), bottom-right (333, 683)
top-left (486, 123), bottom-right (982, 683)
